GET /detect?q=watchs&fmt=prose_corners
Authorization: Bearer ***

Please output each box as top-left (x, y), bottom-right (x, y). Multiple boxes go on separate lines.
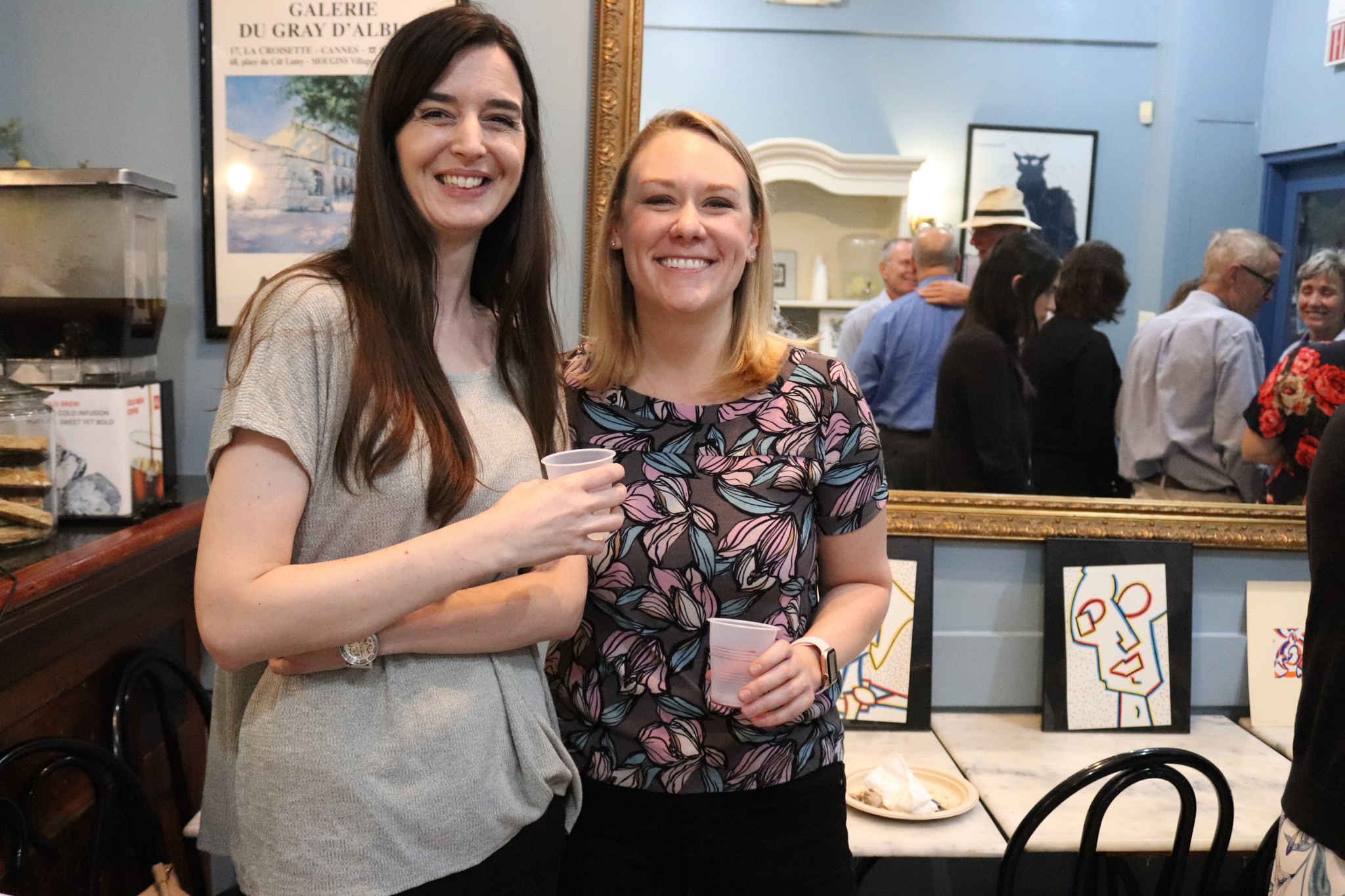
top-left (340, 634), bottom-right (378, 671)
top-left (790, 637), bottom-right (838, 696)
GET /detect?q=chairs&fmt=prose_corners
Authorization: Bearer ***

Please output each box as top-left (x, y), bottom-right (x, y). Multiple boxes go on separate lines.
top-left (0, 648), bottom-right (211, 896)
top-left (996, 747), bottom-right (1234, 896)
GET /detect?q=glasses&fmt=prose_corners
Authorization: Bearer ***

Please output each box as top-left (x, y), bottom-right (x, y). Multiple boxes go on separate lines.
top-left (1239, 263), bottom-right (1276, 297)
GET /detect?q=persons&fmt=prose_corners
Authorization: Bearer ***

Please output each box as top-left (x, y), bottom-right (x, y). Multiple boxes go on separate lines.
top-left (528, 109), bottom-right (893, 896)
top-left (836, 188), bottom-right (1345, 506)
top-left (192, 6), bottom-right (629, 896)
top-left (1264, 404), bottom-right (1345, 896)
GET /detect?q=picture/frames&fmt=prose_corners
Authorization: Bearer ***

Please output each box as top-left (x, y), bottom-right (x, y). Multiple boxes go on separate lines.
top-left (818, 309), bottom-right (850, 356)
top-left (773, 252), bottom-right (795, 300)
top-left (1041, 538), bottom-right (1193, 735)
top-left (198, 0), bottom-right (468, 342)
top-left (957, 124), bottom-right (1098, 283)
top-left (835, 540), bottom-right (936, 730)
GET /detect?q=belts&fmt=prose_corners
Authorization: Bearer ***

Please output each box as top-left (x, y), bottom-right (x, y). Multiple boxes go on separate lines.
top-left (1143, 472), bottom-right (1240, 494)
top-left (877, 422), bottom-right (933, 438)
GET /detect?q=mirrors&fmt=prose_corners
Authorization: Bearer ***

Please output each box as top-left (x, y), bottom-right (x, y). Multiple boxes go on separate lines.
top-left (592, 0), bottom-right (1345, 546)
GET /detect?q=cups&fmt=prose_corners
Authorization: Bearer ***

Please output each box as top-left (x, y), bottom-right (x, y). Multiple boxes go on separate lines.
top-left (707, 617), bottom-right (781, 708)
top-left (541, 449), bottom-right (617, 540)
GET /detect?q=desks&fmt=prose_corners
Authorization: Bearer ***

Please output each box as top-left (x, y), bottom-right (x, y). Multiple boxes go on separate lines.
top-left (842, 713), bottom-right (1292, 858)
top-left (0, 476), bottom-right (210, 883)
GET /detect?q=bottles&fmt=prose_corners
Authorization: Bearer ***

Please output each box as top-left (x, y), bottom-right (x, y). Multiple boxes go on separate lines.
top-left (810, 254), bottom-right (828, 301)
top-left (0, 374), bottom-right (60, 552)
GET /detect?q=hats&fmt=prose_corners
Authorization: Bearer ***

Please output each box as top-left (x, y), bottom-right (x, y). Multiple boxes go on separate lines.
top-left (956, 187), bottom-right (1043, 231)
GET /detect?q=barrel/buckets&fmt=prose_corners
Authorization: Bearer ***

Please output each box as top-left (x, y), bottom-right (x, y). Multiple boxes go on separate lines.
top-left (835, 233), bottom-right (886, 302)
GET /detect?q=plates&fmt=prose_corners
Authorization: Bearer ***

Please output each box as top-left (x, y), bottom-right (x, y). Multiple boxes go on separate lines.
top-left (845, 766), bottom-right (979, 820)
